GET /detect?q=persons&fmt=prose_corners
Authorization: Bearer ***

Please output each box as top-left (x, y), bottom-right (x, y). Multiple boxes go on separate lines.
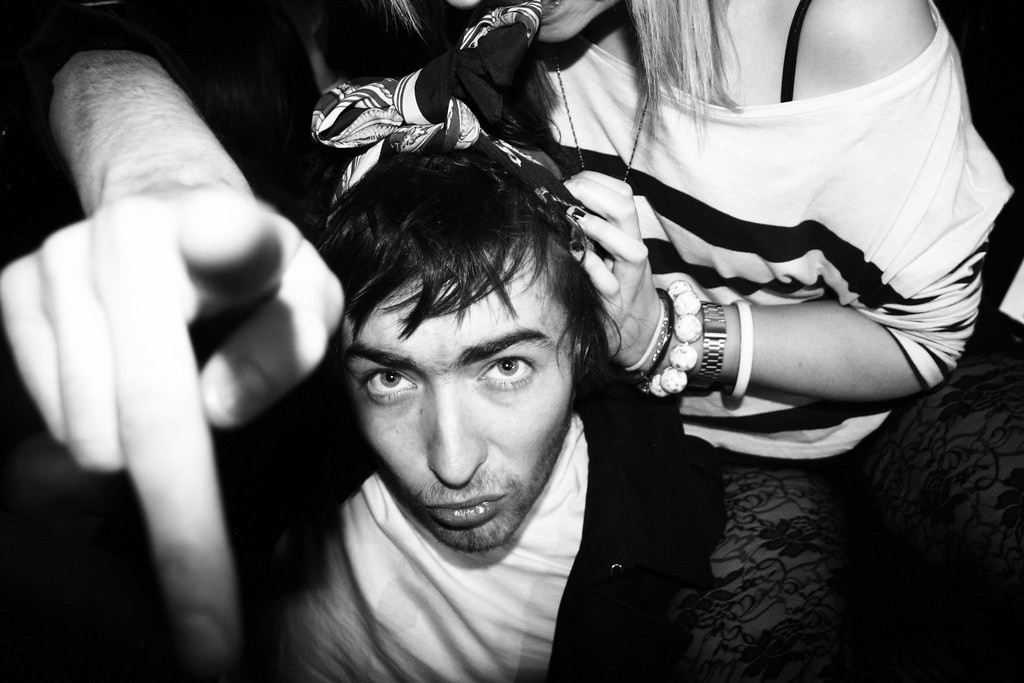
top-left (2, 0), bottom-right (441, 670)
top-left (203, 153), bottom-right (732, 679)
top-left (306, 1), bottom-right (994, 455)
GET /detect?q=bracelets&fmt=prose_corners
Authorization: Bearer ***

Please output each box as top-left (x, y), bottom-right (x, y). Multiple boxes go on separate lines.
top-left (628, 285), bottom-right (756, 397)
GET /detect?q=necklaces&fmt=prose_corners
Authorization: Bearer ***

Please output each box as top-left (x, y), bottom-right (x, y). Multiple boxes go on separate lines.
top-left (544, 42), bottom-right (657, 188)
